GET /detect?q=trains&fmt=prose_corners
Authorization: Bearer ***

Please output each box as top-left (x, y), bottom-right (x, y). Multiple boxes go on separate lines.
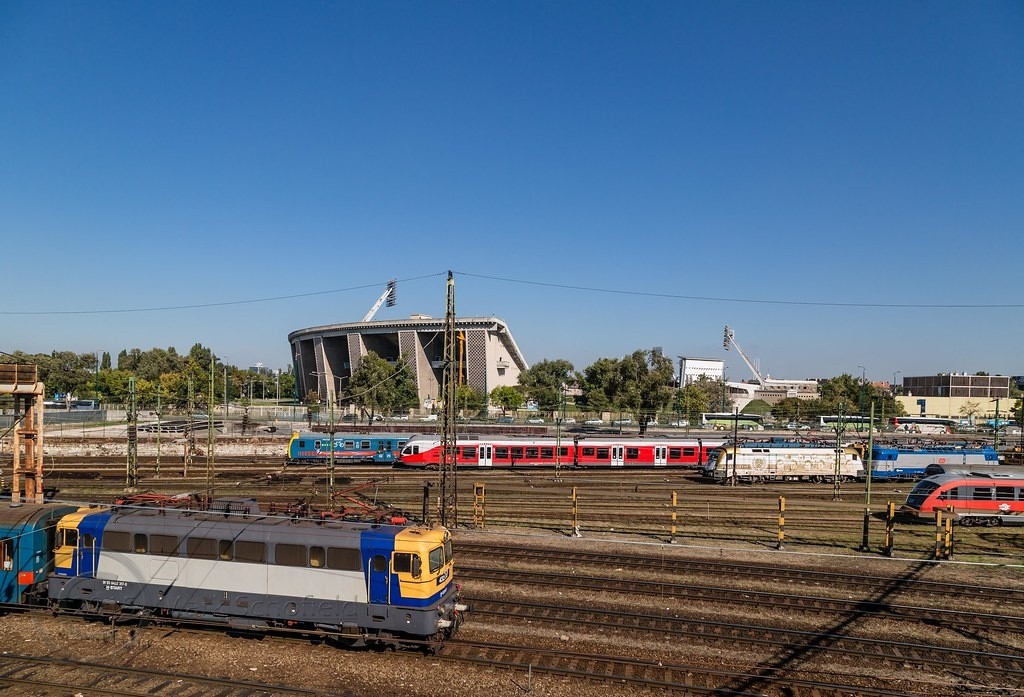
top-left (1, 499), bottom-right (469, 652)
top-left (399, 434), bottom-right (723, 471)
top-left (701, 443), bottom-right (999, 485)
top-left (902, 472), bottom-right (1024, 527)
top-left (288, 430), bottom-right (410, 466)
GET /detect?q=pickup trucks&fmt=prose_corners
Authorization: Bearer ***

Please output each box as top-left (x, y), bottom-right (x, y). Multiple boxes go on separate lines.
top-left (419, 415), bottom-right (438, 422)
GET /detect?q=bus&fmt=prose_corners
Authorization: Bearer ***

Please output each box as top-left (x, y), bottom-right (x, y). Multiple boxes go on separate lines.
top-left (815, 416), bottom-right (881, 434)
top-left (698, 413), bottom-right (765, 432)
top-left (888, 418), bottom-right (957, 434)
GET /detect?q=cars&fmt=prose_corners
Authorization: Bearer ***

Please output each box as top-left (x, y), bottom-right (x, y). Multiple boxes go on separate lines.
top-left (302, 414), bottom-right (322, 422)
top-left (786, 422), bottom-right (811, 431)
top-left (639, 418), bottom-right (659, 427)
top-left (613, 418), bottom-right (632, 426)
top-left (342, 414), bottom-right (408, 423)
top-left (669, 420), bottom-right (691, 429)
top-left (457, 417), bottom-right (602, 426)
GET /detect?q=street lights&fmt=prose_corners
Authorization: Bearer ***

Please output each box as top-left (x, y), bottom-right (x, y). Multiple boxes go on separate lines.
top-left (309, 371), bottom-right (348, 409)
top-left (893, 371), bottom-right (900, 396)
top-left (990, 399), bottom-right (998, 451)
top-left (858, 366), bottom-right (866, 432)
